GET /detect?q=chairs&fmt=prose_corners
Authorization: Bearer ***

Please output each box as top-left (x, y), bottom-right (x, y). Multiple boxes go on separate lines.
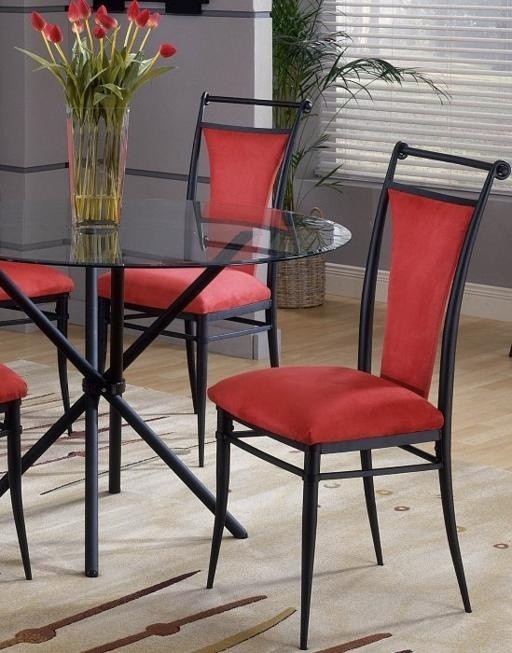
top-left (97, 81), bottom-right (313, 472)
top-left (1, 255), bottom-right (78, 430)
top-left (0, 359), bottom-right (35, 579)
top-left (206, 139), bottom-right (509, 652)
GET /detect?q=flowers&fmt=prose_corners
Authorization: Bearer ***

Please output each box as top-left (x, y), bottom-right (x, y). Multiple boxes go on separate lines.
top-left (7, 0), bottom-right (185, 105)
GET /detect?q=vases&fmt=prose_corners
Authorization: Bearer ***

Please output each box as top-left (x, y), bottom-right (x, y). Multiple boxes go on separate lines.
top-left (61, 100), bottom-right (131, 235)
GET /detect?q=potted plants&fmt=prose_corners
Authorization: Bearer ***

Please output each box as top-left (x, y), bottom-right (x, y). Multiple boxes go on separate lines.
top-left (270, 0), bottom-right (440, 312)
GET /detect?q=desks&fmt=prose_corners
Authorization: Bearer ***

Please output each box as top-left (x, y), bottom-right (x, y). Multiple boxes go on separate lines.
top-left (0, 198), bottom-right (350, 576)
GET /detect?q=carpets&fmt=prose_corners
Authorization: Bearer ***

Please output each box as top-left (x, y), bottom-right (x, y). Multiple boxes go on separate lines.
top-left (2, 347), bottom-right (511, 650)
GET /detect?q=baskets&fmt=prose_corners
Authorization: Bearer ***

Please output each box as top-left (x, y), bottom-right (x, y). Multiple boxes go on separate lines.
top-left (277, 206), bottom-right (334, 308)
